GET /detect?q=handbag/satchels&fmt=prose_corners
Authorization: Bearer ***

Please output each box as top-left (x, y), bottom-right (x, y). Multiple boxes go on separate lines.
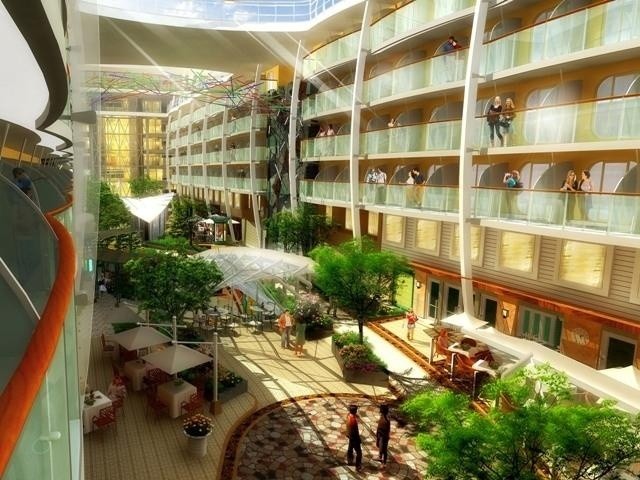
top-left (412, 315), bottom-right (419, 322)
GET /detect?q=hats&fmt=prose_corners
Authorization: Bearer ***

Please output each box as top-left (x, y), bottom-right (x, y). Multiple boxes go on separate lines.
top-left (408, 307), bottom-right (414, 312)
